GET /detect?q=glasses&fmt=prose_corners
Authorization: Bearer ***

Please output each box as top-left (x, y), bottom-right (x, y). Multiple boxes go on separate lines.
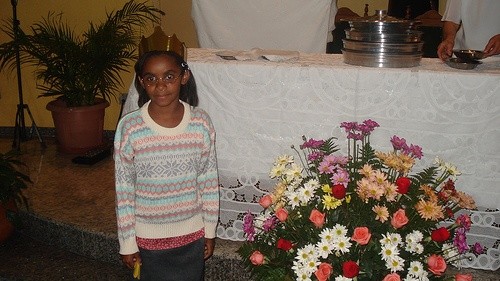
top-left (140, 68), bottom-right (186, 87)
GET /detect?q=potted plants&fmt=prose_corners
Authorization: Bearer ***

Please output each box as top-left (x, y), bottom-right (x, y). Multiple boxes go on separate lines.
top-left (0, 145), bottom-right (33, 242)
top-left (0, 0), bottom-right (166, 156)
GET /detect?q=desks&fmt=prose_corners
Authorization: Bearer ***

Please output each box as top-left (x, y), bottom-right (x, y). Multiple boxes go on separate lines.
top-left (120, 48), bottom-right (500, 270)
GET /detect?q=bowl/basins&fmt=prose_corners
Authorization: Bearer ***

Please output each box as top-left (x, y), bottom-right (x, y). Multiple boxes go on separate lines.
top-left (339, 9), bottom-right (425, 68)
top-left (452, 49), bottom-right (483, 58)
top-left (443, 59), bottom-right (483, 70)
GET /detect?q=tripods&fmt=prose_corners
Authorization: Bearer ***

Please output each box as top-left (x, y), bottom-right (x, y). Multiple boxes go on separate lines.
top-left (11, 0), bottom-right (45, 151)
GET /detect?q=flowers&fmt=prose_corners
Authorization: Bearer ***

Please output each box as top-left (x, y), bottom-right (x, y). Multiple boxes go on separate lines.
top-left (238, 119), bottom-right (485, 281)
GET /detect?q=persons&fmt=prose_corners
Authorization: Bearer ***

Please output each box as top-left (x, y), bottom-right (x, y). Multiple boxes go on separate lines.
top-left (114, 26), bottom-right (219, 280)
top-left (437, 1), bottom-right (499, 60)
